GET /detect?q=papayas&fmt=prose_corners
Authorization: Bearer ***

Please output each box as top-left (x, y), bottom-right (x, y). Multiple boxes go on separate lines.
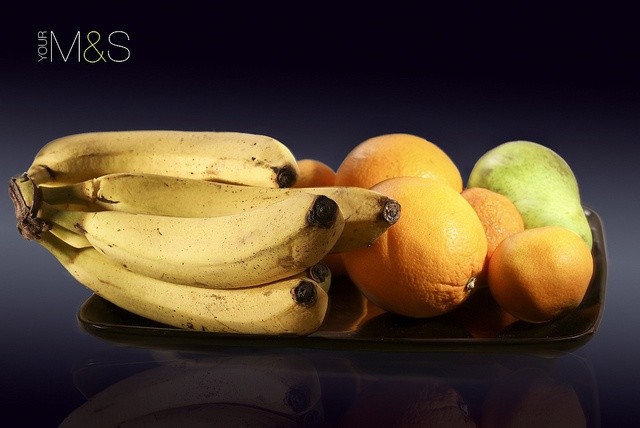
top-left (465, 139), bottom-right (593, 243)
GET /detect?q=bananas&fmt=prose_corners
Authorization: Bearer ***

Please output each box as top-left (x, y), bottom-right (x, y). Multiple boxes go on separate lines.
top-left (7, 128), bottom-right (400, 336)
